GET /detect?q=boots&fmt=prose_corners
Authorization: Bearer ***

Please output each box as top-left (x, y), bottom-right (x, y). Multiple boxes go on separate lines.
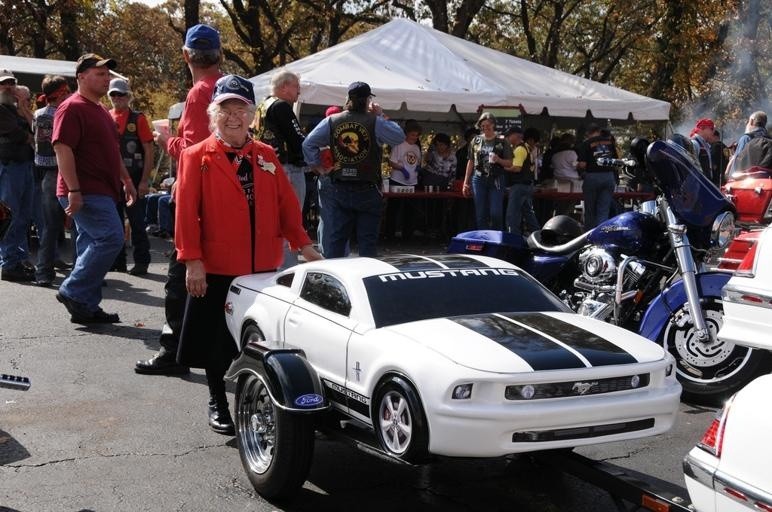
top-left (206, 369), bottom-right (235, 433)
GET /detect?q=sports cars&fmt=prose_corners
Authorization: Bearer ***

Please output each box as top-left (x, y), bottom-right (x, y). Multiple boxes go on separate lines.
top-left (219, 247), bottom-right (682, 461)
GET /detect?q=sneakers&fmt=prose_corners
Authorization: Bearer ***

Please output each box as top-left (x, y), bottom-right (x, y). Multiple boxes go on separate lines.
top-left (55, 292), bottom-right (121, 324)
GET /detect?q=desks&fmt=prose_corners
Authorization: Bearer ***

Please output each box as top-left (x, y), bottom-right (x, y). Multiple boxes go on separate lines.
top-left (381, 190), bottom-right (657, 254)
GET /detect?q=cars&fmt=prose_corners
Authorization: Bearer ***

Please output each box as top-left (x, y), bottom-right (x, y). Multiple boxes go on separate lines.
top-left (681, 163), bottom-right (772, 511)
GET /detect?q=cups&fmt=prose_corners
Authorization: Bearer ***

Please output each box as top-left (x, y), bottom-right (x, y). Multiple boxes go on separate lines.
top-left (151, 118), bottom-right (170, 136)
top-left (382, 176), bottom-right (441, 194)
top-left (488, 152), bottom-right (496, 164)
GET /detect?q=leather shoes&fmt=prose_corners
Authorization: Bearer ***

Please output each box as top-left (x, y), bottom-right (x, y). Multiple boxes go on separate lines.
top-left (134, 352), bottom-right (190, 375)
top-left (0, 260), bottom-right (72, 287)
top-left (109, 264), bottom-right (149, 276)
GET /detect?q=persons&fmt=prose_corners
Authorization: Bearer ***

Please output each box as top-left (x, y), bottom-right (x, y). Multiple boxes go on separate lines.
top-left (252, 73), bottom-right (772, 298)
top-left (2, 50), bottom-right (151, 330)
top-left (172, 74), bottom-right (328, 439)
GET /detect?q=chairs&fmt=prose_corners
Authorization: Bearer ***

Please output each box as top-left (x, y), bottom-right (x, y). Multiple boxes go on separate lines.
top-left (304, 170), bottom-right (321, 230)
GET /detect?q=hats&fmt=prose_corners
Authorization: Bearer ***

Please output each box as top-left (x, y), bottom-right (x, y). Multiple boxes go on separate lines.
top-left (75, 53), bottom-right (119, 78)
top-left (185, 24), bottom-right (221, 50)
top-left (1, 68), bottom-right (18, 83)
top-left (107, 79), bottom-right (130, 94)
top-left (348, 82), bottom-right (376, 97)
top-left (211, 74), bottom-right (256, 105)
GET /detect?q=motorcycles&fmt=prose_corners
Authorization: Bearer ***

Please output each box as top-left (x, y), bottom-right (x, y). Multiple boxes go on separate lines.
top-left (441, 116), bottom-right (761, 403)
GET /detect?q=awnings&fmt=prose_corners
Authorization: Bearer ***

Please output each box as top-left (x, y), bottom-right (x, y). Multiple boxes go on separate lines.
top-left (168, 19), bottom-right (670, 142)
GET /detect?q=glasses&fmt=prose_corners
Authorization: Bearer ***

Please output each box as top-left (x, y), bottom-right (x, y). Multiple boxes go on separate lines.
top-left (109, 93), bottom-right (126, 96)
